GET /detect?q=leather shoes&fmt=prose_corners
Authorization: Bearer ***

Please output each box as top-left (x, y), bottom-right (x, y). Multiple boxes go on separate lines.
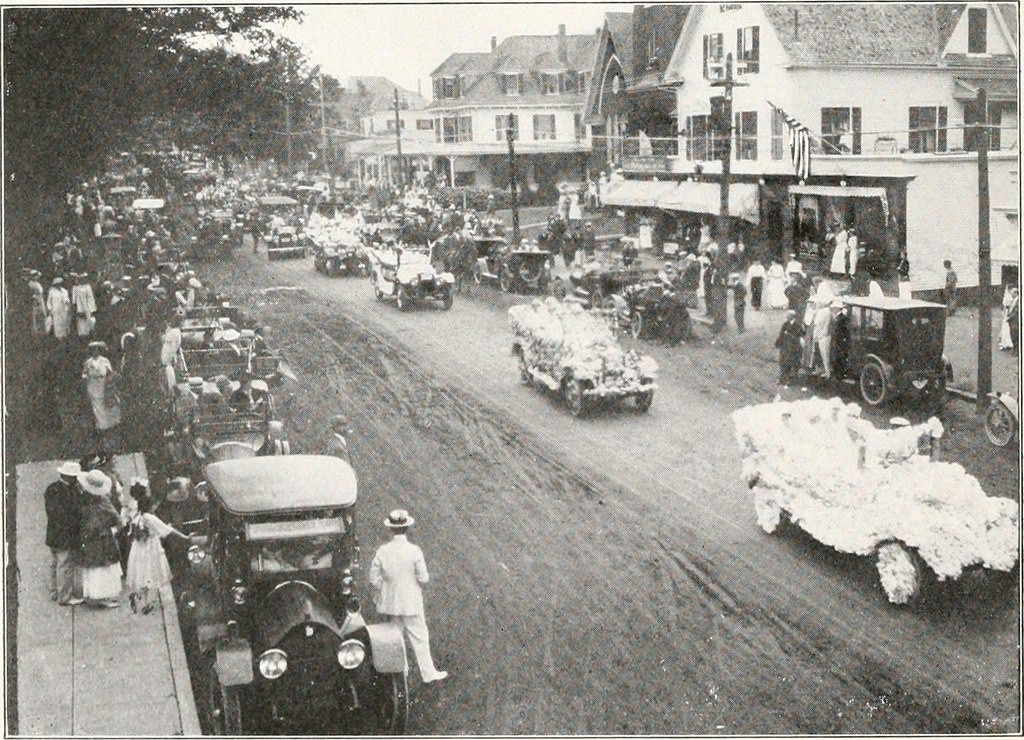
top-left (52, 595), bottom-right (85, 606)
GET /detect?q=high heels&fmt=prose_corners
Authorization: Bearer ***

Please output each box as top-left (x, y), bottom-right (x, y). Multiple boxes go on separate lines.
top-left (98, 598), bottom-right (121, 608)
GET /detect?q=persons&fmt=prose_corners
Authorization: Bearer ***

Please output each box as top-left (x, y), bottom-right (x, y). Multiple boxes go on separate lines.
top-left (537, 163), bottom-right (1020, 385)
top-left (27, 148), bottom-right (351, 615)
top-left (368, 508), bottom-right (448, 683)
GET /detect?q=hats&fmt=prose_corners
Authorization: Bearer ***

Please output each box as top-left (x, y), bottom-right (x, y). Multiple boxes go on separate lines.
top-left (57, 461), bottom-right (82, 476)
top-left (202, 329), bottom-right (215, 338)
top-left (222, 328), bottom-right (241, 342)
top-left (329, 415), bottom-right (348, 429)
top-left (52, 277), bottom-right (63, 286)
top-left (729, 273), bottom-right (739, 278)
top-left (187, 375), bottom-right (208, 390)
top-left (214, 375), bottom-right (233, 387)
top-left (383, 509), bottom-right (415, 528)
top-left (77, 469), bottom-right (112, 496)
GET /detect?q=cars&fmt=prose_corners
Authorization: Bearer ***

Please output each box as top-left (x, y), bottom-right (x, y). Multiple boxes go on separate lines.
top-left (98, 135), bottom-right (958, 469)
top-left (370, 249), bottom-right (459, 310)
top-left (731, 396), bottom-right (1020, 611)
top-left (504, 297), bottom-right (661, 417)
top-left (177, 452), bottom-right (410, 737)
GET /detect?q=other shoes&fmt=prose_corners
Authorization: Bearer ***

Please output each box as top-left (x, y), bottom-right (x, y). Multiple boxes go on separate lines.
top-left (130, 602), bottom-right (137, 612)
top-left (429, 670), bottom-right (448, 683)
top-left (142, 604), bottom-right (154, 614)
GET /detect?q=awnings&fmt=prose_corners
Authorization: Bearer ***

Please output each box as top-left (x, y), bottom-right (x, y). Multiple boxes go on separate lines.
top-left (601, 180), bottom-right (678, 208)
top-left (659, 182), bottom-right (759, 225)
